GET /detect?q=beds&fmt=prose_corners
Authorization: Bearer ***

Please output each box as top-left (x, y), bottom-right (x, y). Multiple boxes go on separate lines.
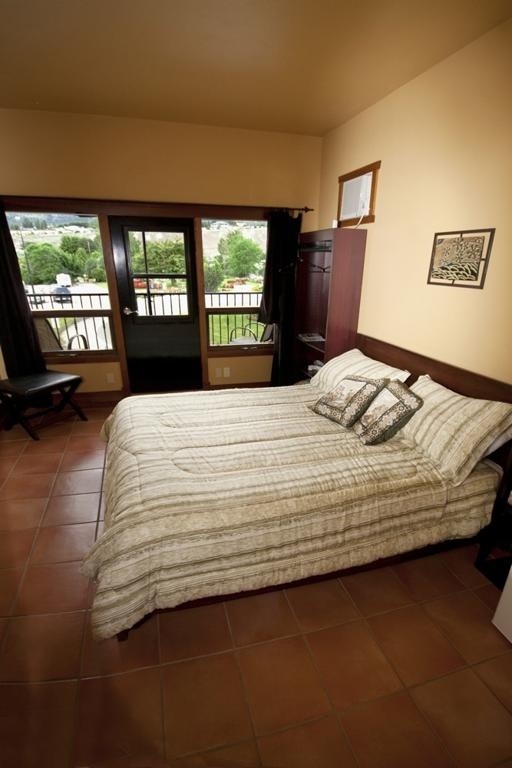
top-left (97, 331), bottom-right (511, 641)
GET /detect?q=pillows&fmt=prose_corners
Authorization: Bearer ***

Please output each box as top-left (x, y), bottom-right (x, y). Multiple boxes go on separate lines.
top-left (306, 346), bottom-right (511, 493)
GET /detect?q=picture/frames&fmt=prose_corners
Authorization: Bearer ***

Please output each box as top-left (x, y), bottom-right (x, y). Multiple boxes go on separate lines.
top-left (426, 228), bottom-right (496, 289)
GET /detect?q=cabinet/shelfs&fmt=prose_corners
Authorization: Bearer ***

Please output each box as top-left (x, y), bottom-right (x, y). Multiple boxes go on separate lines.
top-left (293, 229), bottom-right (368, 377)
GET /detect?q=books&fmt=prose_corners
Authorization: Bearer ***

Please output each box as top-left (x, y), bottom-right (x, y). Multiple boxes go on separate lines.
top-left (297, 331), bottom-right (325, 343)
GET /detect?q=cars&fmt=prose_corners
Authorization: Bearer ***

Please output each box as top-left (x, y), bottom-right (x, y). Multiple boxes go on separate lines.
top-left (134, 277), bottom-right (162, 289)
top-left (51, 286), bottom-right (71, 303)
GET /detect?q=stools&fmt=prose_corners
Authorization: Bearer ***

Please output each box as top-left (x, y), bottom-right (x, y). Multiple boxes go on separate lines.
top-left (0, 368), bottom-right (88, 442)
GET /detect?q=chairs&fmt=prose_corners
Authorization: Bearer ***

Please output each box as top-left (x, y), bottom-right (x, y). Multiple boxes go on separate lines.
top-left (34, 314), bottom-right (89, 352)
top-left (230, 320), bottom-right (274, 345)
